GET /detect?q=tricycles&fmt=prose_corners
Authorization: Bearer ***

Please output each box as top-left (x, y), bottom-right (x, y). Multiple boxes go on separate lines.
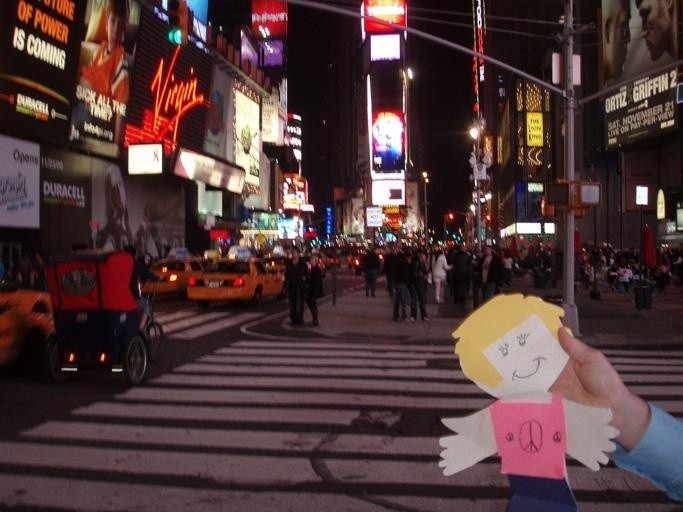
top-left (41, 250), bottom-right (168, 384)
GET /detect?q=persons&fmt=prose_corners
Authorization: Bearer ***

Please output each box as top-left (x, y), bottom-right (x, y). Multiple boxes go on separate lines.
top-left (94, 164), bottom-right (152, 255)
top-left (601, 0), bottom-right (630, 88)
top-left (122, 246), bottom-right (162, 341)
top-left (283, 246), bottom-right (327, 328)
top-left (635, 0), bottom-right (679, 63)
top-left (549, 327), bottom-right (681, 506)
top-left (73, 1), bottom-right (129, 145)
top-left (364, 238), bottom-right (683, 328)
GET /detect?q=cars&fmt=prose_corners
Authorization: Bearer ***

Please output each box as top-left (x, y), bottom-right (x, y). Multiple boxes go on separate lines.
top-left (2, 285), bottom-right (54, 376)
top-left (142, 247), bottom-right (387, 304)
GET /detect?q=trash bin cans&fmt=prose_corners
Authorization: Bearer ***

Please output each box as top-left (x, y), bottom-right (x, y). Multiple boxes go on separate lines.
top-left (634, 288), bottom-right (653, 309)
top-left (535, 273), bottom-right (549, 288)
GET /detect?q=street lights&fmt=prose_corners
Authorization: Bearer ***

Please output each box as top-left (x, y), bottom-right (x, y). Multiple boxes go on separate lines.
top-left (634, 183), bottom-right (652, 309)
top-left (448, 209), bottom-right (474, 247)
top-left (421, 171), bottom-right (432, 250)
